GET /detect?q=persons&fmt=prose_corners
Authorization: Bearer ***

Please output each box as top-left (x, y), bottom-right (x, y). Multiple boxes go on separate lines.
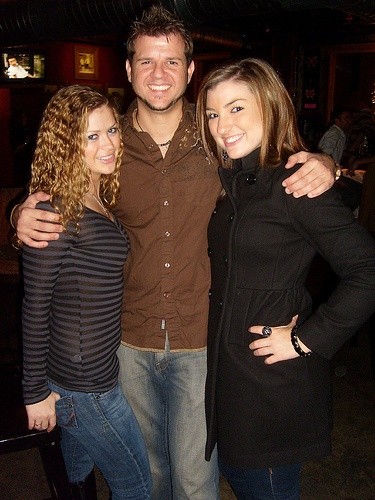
top-left (319, 103), bottom-right (375, 213)
top-left (21, 85), bottom-right (154, 500)
top-left (13, 112), bottom-right (30, 177)
top-left (194, 58), bottom-right (375, 500)
top-left (9, 0), bottom-right (342, 500)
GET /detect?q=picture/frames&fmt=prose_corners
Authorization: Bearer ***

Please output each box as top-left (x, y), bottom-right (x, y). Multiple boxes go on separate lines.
top-left (72, 43), bottom-right (103, 80)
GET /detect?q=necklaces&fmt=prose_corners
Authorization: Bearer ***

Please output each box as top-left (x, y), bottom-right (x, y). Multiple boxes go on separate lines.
top-left (136, 110), bottom-right (171, 146)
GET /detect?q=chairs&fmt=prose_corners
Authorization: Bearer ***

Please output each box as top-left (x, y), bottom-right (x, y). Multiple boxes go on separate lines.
top-left (0, 185), bottom-right (28, 272)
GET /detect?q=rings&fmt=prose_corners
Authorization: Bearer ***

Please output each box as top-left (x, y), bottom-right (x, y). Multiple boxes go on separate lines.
top-left (262, 326), bottom-right (272, 336)
top-left (350, 171), bottom-right (352, 172)
top-left (36, 424), bottom-right (41, 426)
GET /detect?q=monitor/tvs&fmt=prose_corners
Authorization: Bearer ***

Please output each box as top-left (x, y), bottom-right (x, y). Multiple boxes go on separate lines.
top-left (0, 47), bottom-right (49, 83)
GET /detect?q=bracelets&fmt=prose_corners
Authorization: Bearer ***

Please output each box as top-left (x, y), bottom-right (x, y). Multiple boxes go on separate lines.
top-left (25, 143), bottom-right (27, 144)
top-left (291, 326), bottom-right (310, 358)
top-left (335, 164), bottom-right (341, 180)
top-left (10, 204), bottom-right (20, 230)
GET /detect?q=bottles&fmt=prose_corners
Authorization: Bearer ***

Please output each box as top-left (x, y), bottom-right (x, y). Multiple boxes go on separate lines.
top-left (293, 90), bottom-right (296, 101)
top-left (298, 118), bottom-right (308, 135)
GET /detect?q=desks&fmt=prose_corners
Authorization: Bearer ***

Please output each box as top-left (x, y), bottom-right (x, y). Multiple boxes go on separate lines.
top-left (0, 275), bottom-right (80, 500)
